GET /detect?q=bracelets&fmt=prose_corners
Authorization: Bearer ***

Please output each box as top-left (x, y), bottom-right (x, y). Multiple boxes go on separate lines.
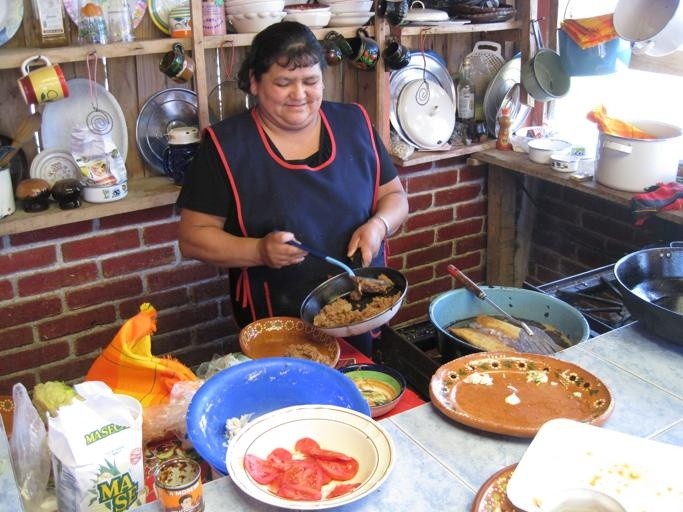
top-left (372, 215), bottom-right (389, 240)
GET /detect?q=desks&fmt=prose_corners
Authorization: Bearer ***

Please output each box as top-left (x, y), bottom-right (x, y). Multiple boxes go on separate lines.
top-left (466, 147), bottom-right (683, 286)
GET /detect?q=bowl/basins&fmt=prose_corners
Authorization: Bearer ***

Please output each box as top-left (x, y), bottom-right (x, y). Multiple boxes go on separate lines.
top-left (427, 285), bottom-right (589, 360)
top-left (77, 179), bottom-right (133, 205)
top-left (334, 361), bottom-right (406, 420)
top-left (512, 123), bottom-right (592, 174)
top-left (225, 0), bottom-right (380, 34)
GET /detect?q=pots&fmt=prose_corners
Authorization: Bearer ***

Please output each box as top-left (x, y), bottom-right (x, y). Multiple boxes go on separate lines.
top-left (630, 1), bottom-right (683, 57)
top-left (297, 247), bottom-right (409, 337)
top-left (614, 240), bottom-right (683, 352)
top-left (522, 17), bottom-right (571, 101)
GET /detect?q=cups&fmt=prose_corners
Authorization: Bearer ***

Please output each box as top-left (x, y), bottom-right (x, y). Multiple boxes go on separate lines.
top-left (16, 54), bottom-right (68, 105)
top-left (0, 167), bottom-right (16, 219)
top-left (161, 125), bottom-right (206, 189)
top-left (320, 0), bottom-right (414, 72)
top-left (31, 0), bottom-right (226, 48)
top-left (158, 42), bottom-right (196, 84)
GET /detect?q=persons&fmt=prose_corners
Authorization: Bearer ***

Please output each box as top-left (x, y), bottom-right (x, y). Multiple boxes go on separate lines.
top-left (177, 21), bottom-right (409, 359)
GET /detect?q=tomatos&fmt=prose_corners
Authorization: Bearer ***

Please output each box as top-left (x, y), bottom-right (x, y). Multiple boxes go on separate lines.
top-left (244, 438), bottom-right (360, 503)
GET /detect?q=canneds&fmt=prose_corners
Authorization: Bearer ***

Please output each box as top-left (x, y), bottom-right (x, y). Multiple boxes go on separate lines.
top-left (154, 456), bottom-right (205, 512)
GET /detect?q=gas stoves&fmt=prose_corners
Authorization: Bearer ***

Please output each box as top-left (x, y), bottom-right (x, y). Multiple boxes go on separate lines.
top-left (381, 248), bottom-right (638, 406)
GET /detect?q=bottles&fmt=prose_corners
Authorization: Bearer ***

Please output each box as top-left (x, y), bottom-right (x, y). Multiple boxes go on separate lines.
top-left (455, 55), bottom-right (474, 125)
top-left (496, 108), bottom-right (513, 151)
top-left (16, 178), bottom-right (50, 214)
top-left (465, 118), bottom-right (489, 144)
top-left (51, 179), bottom-right (85, 210)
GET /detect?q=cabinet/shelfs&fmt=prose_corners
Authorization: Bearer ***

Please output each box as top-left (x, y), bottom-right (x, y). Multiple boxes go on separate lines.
top-left (0, 0), bottom-right (538, 237)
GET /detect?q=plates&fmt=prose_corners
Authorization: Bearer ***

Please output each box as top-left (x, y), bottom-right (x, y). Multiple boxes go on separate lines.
top-left (238, 315), bottom-right (342, 372)
top-left (474, 462), bottom-right (527, 512)
top-left (228, 404), bottom-right (394, 511)
top-left (612, 0), bottom-right (679, 41)
top-left (185, 357), bottom-right (373, 481)
top-left (29, 148), bottom-right (82, 202)
top-left (414, 3), bottom-right (517, 26)
top-left (0, 0), bottom-right (26, 51)
top-left (40, 78), bottom-right (128, 179)
top-left (504, 419), bottom-right (680, 512)
top-left (432, 351), bottom-right (618, 436)
